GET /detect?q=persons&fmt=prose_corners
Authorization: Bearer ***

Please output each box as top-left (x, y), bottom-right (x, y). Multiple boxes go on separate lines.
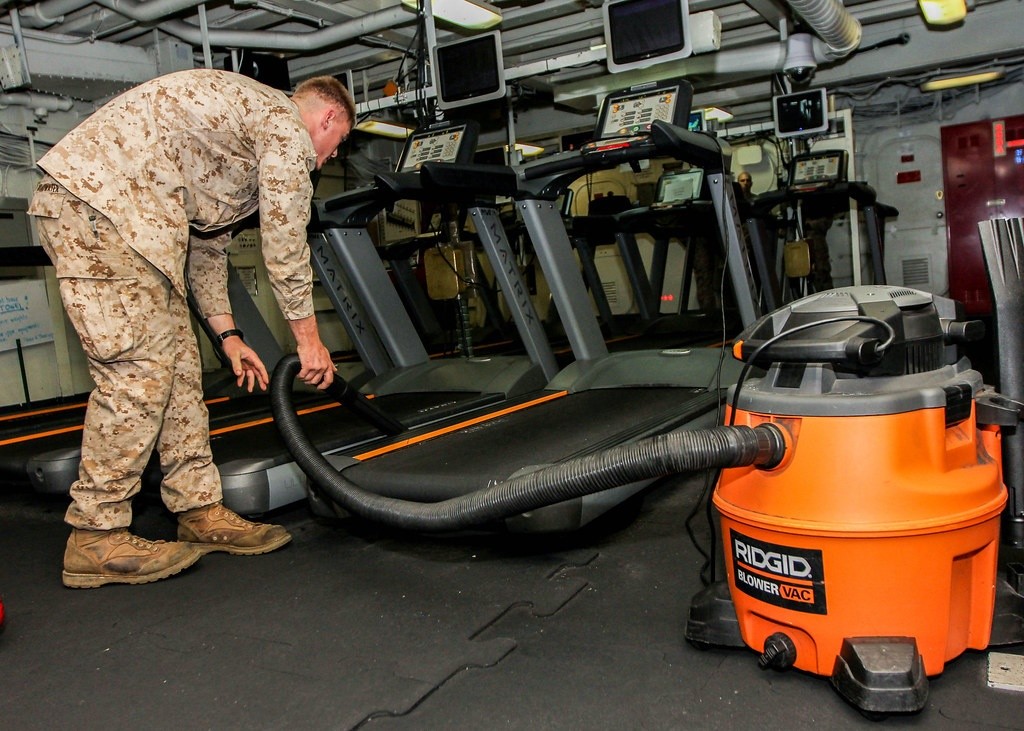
top-left (738, 172), bottom-right (758, 203)
top-left (26, 68), bottom-right (356, 589)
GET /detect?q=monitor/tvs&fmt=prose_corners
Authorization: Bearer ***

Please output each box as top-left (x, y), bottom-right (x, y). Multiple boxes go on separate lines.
top-left (793, 155), bottom-right (840, 181)
top-left (399, 124), bottom-right (466, 173)
top-left (599, 85), bottom-right (678, 139)
top-left (433, 29), bottom-right (506, 110)
top-left (654, 168), bottom-right (704, 203)
top-left (332, 69), bottom-right (355, 103)
top-left (602, 0), bottom-right (693, 73)
top-left (773, 87), bottom-right (829, 138)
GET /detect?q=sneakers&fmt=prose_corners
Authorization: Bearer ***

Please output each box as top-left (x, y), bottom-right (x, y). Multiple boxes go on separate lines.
top-left (62, 527), bottom-right (202, 589)
top-left (176, 500), bottom-right (293, 556)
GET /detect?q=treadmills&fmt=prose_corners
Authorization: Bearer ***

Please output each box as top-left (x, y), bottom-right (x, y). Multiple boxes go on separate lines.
top-left (287, 0), bottom-right (763, 535)
top-left (0, 68), bottom-right (394, 493)
top-left (132, 31), bottom-right (558, 514)
top-left (427, 86), bottom-right (877, 370)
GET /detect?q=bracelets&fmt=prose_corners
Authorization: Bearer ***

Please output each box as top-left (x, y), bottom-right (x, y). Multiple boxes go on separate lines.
top-left (216, 328), bottom-right (243, 346)
